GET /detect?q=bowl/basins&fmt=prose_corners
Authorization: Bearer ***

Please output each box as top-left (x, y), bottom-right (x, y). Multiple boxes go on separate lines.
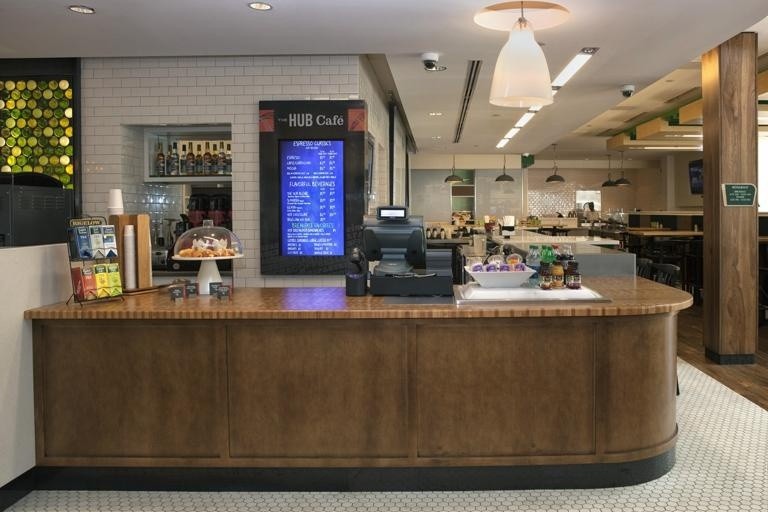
top-left (463, 264), bottom-right (535, 288)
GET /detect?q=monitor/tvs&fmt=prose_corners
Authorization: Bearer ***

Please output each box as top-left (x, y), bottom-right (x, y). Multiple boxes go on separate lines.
top-left (688, 160), bottom-right (702, 194)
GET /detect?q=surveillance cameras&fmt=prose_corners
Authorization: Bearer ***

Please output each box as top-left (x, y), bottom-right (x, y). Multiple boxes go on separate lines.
top-left (421, 53), bottom-right (440, 69)
top-left (620, 85), bottom-right (635, 98)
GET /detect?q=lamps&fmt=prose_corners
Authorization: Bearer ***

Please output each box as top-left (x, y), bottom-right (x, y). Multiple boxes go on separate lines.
top-left (444, 150), bottom-right (632, 189)
top-left (473, 1), bottom-right (571, 108)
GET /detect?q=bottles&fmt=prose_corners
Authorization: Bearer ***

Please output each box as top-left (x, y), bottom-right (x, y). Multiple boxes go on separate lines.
top-left (155, 141), bottom-right (231, 176)
top-left (526, 244), bottom-right (581, 290)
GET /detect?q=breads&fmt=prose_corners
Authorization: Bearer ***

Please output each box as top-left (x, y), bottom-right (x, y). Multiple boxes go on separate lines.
top-left (179, 247), bottom-right (235, 257)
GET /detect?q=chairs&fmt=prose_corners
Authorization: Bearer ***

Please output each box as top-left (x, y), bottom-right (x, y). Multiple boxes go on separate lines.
top-left (649, 262), bottom-right (681, 395)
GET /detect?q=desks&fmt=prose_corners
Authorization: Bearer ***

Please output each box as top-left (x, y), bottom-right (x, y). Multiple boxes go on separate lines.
top-left (534, 211), bottom-right (704, 295)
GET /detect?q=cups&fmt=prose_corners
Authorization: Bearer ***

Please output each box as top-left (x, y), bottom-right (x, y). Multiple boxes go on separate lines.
top-left (427, 228), bottom-right (444, 239)
top-left (107, 189), bottom-right (124, 217)
top-left (123, 224), bottom-right (135, 291)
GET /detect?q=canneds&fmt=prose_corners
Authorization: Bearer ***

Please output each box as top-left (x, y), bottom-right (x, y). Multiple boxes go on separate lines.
top-left (538, 261), bottom-right (581, 290)
